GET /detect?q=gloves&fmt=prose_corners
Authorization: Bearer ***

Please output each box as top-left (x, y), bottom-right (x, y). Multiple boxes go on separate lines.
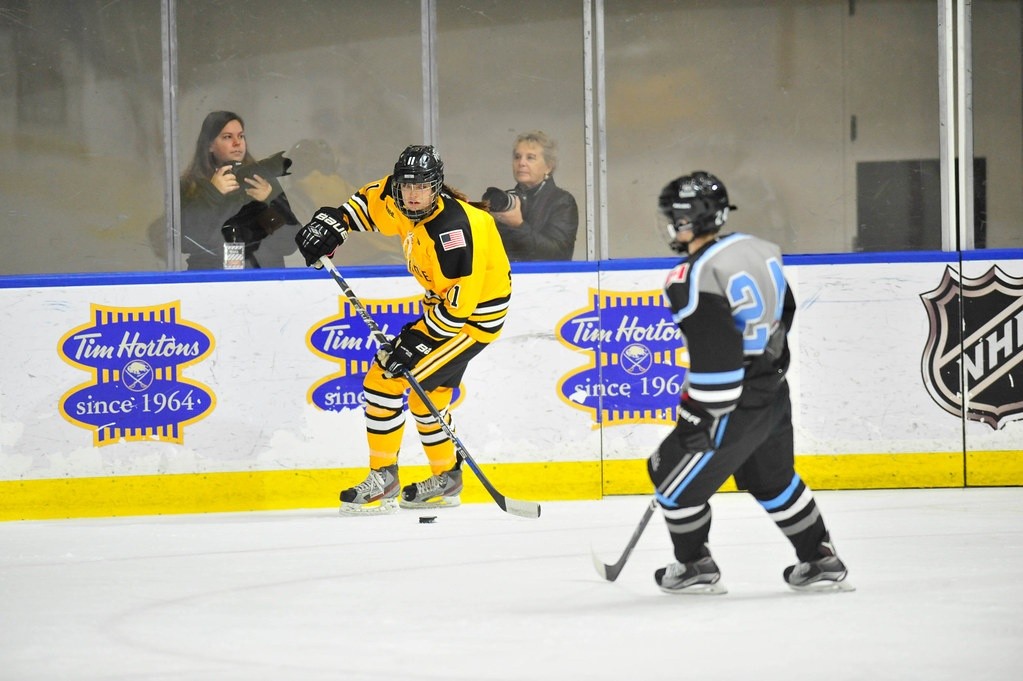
top-left (676, 392), bottom-right (716, 452)
top-left (295, 207), bottom-right (349, 270)
top-left (374, 323), bottom-right (442, 379)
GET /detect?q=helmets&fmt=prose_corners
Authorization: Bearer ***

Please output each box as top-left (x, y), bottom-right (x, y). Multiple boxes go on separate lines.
top-left (391, 145), bottom-right (445, 221)
top-left (655, 171), bottom-right (737, 255)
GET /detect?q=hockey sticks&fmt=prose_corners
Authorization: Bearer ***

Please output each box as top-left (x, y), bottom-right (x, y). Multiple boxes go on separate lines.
top-left (319, 254), bottom-right (542, 520)
top-left (584, 496), bottom-right (660, 583)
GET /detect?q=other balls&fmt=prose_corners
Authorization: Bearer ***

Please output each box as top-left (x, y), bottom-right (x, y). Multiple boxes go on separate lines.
top-left (418, 517), bottom-right (438, 524)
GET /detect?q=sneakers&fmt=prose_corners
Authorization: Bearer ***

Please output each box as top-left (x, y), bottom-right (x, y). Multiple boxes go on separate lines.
top-left (340, 464), bottom-right (400, 516)
top-left (655, 546), bottom-right (728, 594)
top-left (399, 450), bottom-right (464, 509)
top-left (783, 531), bottom-right (856, 592)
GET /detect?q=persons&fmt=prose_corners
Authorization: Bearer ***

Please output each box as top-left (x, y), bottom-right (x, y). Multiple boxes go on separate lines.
top-left (646, 170), bottom-right (858, 597)
top-left (295, 143), bottom-right (512, 516)
top-left (282, 138), bottom-right (406, 266)
top-left (490, 131), bottom-right (579, 262)
top-left (163, 110), bottom-right (302, 271)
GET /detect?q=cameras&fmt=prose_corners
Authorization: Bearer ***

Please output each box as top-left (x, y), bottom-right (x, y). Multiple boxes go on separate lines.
top-left (483, 186), bottom-right (529, 217)
top-left (220, 150), bottom-right (292, 203)
